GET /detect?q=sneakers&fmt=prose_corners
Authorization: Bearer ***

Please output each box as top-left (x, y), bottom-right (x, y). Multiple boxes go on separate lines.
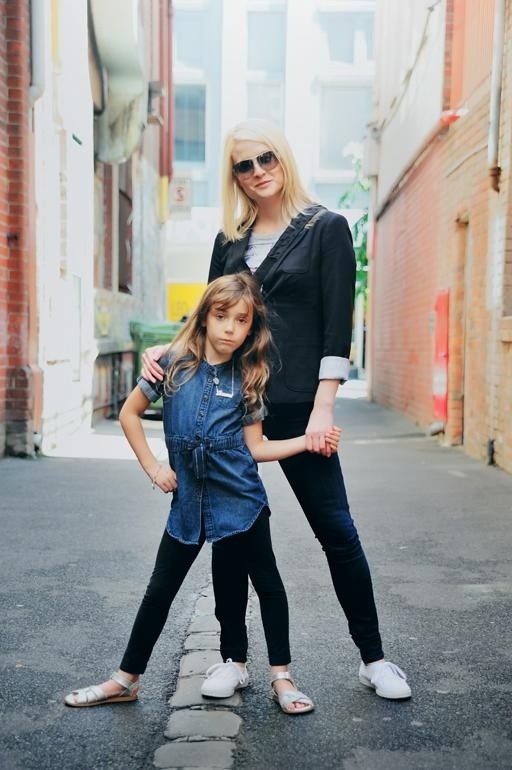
top-left (359, 660), bottom-right (411, 698)
top-left (202, 658), bottom-right (249, 698)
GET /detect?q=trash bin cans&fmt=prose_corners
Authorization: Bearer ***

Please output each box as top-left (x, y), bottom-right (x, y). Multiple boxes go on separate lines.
top-left (130, 321), bottom-right (185, 421)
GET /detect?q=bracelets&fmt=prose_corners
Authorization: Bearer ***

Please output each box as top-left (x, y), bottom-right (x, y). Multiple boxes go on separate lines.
top-left (151, 464), bottom-right (163, 491)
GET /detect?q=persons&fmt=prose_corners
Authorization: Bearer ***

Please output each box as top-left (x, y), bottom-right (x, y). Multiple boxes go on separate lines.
top-left (61, 268), bottom-right (343, 716)
top-left (139, 120), bottom-right (415, 705)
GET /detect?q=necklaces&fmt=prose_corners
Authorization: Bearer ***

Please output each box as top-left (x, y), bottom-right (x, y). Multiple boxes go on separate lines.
top-left (207, 355), bottom-right (235, 399)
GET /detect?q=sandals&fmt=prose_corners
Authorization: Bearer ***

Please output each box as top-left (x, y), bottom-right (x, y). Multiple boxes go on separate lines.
top-left (270, 669), bottom-right (315, 714)
top-left (64, 672), bottom-right (140, 707)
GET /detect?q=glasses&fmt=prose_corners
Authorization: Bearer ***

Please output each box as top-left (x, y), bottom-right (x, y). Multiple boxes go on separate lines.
top-left (232, 150), bottom-right (278, 182)
